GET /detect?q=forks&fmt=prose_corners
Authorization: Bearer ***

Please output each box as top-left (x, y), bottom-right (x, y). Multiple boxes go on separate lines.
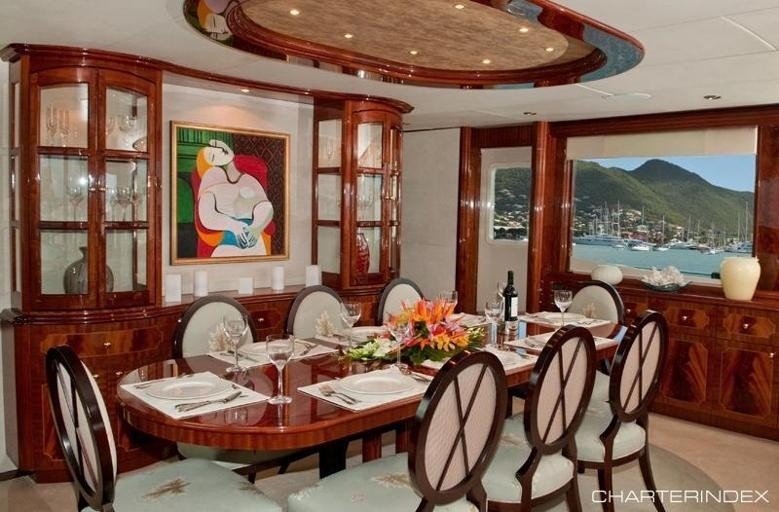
top-left (317, 381), bottom-right (367, 407)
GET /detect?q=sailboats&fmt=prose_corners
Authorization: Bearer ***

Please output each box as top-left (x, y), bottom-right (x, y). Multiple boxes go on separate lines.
top-left (573, 200), bottom-right (754, 254)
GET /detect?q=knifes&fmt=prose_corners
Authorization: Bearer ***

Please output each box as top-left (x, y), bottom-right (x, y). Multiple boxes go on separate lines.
top-left (175, 394), bottom-right (248, 408)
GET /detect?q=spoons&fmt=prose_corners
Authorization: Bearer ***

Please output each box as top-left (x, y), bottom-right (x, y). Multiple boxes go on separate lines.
top-left (400, 368), bottom-right (431, 383)
top-left (180, 391), bottom-right (242, 415)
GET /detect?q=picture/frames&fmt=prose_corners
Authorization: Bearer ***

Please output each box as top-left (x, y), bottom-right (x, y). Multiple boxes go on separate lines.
top-left (486, 162), bottom-right (532, 246)
top-left (169, 119), bottom-right (291, 266)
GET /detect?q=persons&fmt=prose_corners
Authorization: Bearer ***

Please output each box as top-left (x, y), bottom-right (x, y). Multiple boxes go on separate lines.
top-left (190, 139), bottom-right (277, 259)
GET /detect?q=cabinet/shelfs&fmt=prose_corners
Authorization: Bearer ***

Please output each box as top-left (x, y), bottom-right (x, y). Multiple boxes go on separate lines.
top-left (1, 43), bottom-right (416, 485)
top-left (557, 284), bottom-right (778, 442)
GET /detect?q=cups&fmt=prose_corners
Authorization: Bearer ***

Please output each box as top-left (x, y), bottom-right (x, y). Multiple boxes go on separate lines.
top-left (440, 290), bottom-right (461, 309)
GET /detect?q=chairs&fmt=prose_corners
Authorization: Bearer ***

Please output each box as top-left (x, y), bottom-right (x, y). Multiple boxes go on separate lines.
top-left (574, 308), bottom-right (669, 511)
top-left (482, 324), bottom-right (597, 512)
top-left (506, 280), bottom-right (626, 418)
top-left (174, 293), bottom-right (294, 483)
top-left (45, 346), bottom-right (284, 512)
top-left (286, 285), bottom-right (352, 340)
top-left (287, 347), bottom-right (509, 512)
top-left (375, 277), bottom-right (423, 325)
top-left (719, 257), bottom-right (762, 302)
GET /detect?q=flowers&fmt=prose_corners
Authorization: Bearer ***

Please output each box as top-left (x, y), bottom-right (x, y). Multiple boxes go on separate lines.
top-left (345, 292), bottom-right (488, 367)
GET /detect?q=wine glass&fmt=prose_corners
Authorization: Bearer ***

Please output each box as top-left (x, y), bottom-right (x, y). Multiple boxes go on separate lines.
top-left (483, 299), bottom-right (504, 349)
top-left (336, 298), bottom-right (362, 354)
top-left (496, 279), bottom-right (509, 298)
top-left (385, 314), bottom-right (410, 371)
top-left (219, 315), bottom-right (248, 374)
top-left (105, 184), bottom-right (143, 222)
top-left (334, 187), bottom-right (374, 221)
top-left (555, 289), bottom-right (571, 327)
top-left (66, 186), bottom-right (83, 222)
top-left (105, 113), bottom-right (136, 149)
top-left (262, 332), bottom-right (300, 404)
top-left (325, 138), bottom-right (334, 167)
top-left (46, 105), bottom-right (70, 147)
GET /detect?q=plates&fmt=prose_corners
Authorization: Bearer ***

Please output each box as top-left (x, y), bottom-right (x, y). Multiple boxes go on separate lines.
top-left (544, 313), bottom-right (588, 323)
top-left (146, 379), bottom-right (228, 401)
top-left (340, 372), bottom-right (417, 394)
top-left (241, 341), bottom-right (309, 355)
top-left (341, 323), bottom-right (384, 340)
top-left (639, 276), bottom-right (692, 291)
top-left (488, 350), bottom-right (521, 367)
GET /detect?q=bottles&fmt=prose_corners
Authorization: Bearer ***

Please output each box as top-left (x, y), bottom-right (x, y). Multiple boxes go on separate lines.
top-left (503, 322), bottom-right (519, 352)
top-left (502, 270), bottom-right (518, 323)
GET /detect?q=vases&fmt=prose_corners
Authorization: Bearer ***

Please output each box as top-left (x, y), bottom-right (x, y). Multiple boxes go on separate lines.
top-left (356, 232), bottom-right (369, 285)
top-left (64, 246), bottom-right (114, 294)
